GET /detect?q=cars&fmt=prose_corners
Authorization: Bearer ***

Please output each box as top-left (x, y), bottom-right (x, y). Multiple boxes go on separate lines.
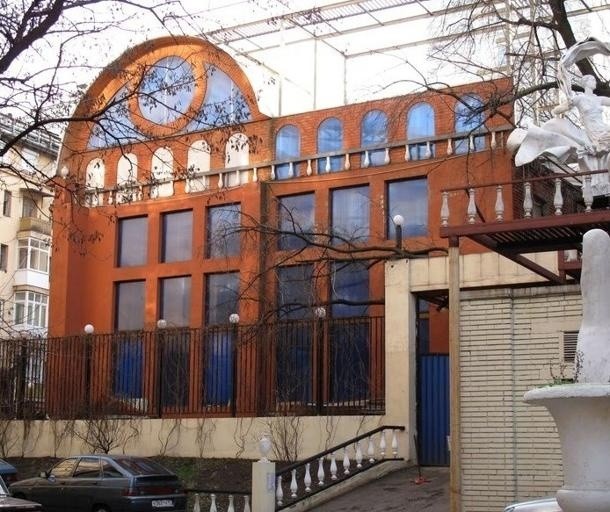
top-left (0, 475), bottom-right (42, 512)
top-left (8, 454), bottom-right (187, 511)
top-left (0, 458), bottom-right (17, 488)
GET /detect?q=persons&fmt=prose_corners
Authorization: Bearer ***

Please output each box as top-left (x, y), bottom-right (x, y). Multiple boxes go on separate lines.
top-left (553, 75), bottom-right (610, 155)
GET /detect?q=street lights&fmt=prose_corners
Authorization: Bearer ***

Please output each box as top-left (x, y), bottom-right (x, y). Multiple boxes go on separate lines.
top-left (313, 307), bottom-right (330, 416)
top-left (18, 331), bottom-right (29, 419)
top-left (83, 323), bottom-right (95, 419)
top-left (228, 311), bottom-right (241, 417)
top-left (156, 318), bottom-right (167, 419)
top-left (393, 214), bottom-right (404, 260)
